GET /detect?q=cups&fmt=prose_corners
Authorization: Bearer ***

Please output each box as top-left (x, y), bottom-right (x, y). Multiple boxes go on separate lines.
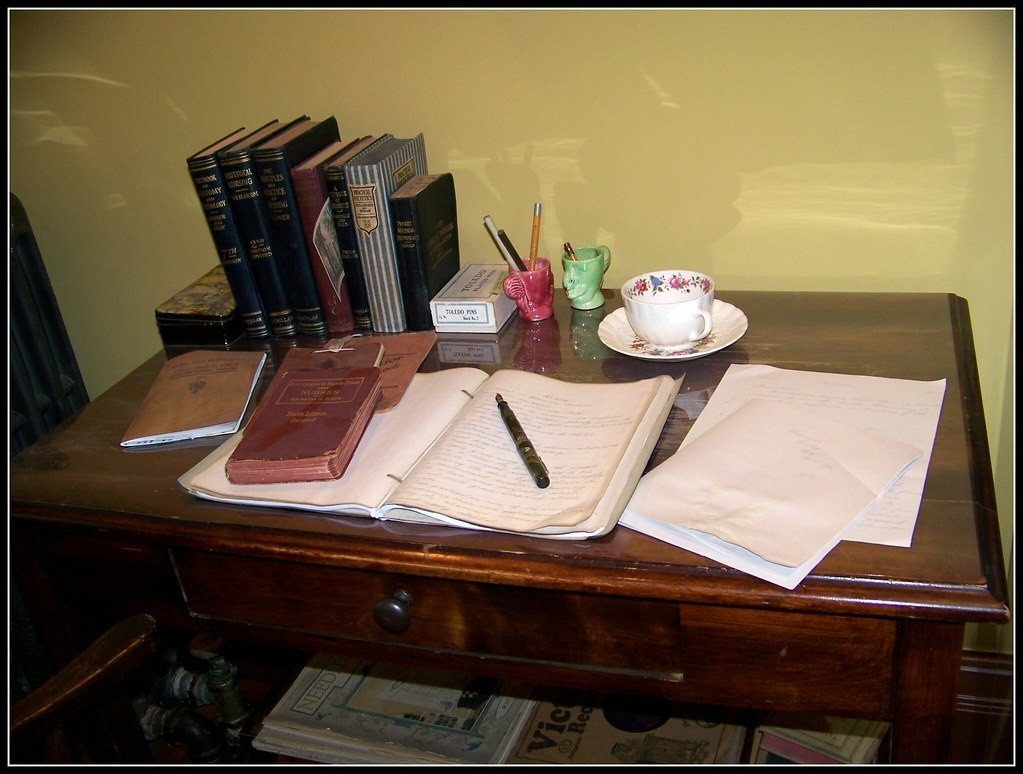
top-left (621, 270), bottom-right (715, 347)
top-left (562, 246), bottom-right (611, 310)
top-left (503, 257), bottom-right (554, 322)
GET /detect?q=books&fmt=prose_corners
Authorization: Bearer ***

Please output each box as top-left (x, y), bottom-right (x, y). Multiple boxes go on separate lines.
top-left (618, 396), bottom-right (923, 591)
top-left (322, 132), bottom-right (388, 330)
top-left (342, 132), bottom-right (429, 335)
top-left (290, 136), bottom-right (361, 334)
top-left (243, 341), bottom-right (385, 436)
top-left (216, 114), bottom-right (305, 336)
top-left (504, 700), bottom-right (745, 765)
top-left (178, 366), bottom-right (687, 544)
top-left (390, 170), bottom-right (460, 333)
top-left (262, 664), bottom-right (535, 765)
top-left (186, 118), bottom-right (278, 338)
top-left (251, 726), bottom-right (389, 764)
top-left (250, 112), bottom-right (341, 334)
top-left (224, 368), bottom-right (384, 487)
top-left (321, 329), bottom-right (440, 413)
top-left (119, 348), bottom-right (268, 449)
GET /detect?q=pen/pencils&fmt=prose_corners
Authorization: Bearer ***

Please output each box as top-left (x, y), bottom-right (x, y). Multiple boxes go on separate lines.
top-left (492, 390), bottom-right (551, 488)
top-left (497, 229), bottom-right (528, 271)
top-left (528, 203), bottom-right (542, 270)
top-left (483, 214), bottom-right (519, 271)
top-left (564, 241), bottom-right (577, 261)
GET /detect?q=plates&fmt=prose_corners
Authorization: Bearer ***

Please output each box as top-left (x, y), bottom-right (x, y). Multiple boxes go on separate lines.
top-left (597, 298), bottom-right (749, 362)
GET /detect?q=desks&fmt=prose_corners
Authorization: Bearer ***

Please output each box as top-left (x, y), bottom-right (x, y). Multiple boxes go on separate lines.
top-left (10, 289), bottom-right (1011, 760)
